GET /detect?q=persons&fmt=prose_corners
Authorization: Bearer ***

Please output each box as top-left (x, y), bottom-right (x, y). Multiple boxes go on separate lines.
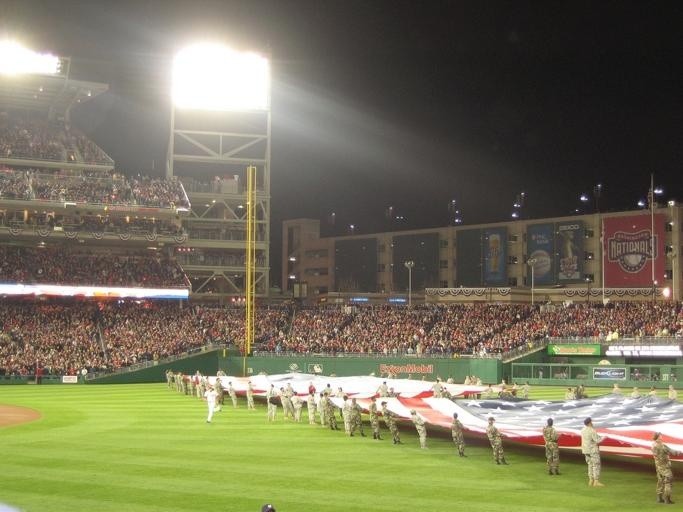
top-left (580, 417), bottom-right (608, 487)
top-left (542, 418), bottom-right (561, 475)
top-left (370, 395), bottom-right (384, 441)
top-left (381, 401), bottom-right (404, 445)
top-left (215, 297), bottom-right (683, 437)
top-left (450, 413), bottom-right (468, 457)
top-left (409, 409), bottom-right (430, 450)
top-left (1, 104), bottom-right (216, 422)
top-left (486, 417), bottom-right (510, 465)
top-left (652, 431), bottom-right (683, 503)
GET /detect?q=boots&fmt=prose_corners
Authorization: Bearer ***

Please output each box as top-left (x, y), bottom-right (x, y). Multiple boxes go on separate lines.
top-left (459, 452), bottom-right (467, 457)
top-left (350, 432), bottom-right (355, 436)
top-left (548, 469), bottom-right (554, 475)
top-left (657, 494), bottom-right (665, 504)
top-left (393, 437), bottom-right (400, 444)
top-left (665, 496), bottom-right (675, 504)
top-left (501, 459), bottom-right (508, 464)
top-left (377, 435), bottom-right (383, 440)
top-left (374, 432), bottom-right (377, 439)
top-left (589, 478), bottom-right (594, 486)
top-left (335, 425), bottom-right (340, 430)
top-left (360, 433), bottom-right (367, 437)
top-left (495, 460), bottom-right (501, 464)
top-left (593, 479), bottom-right (604, 487)
top-left (555, 468), bottom-right (560, 475)
top-left (331, 425), bottom-right (334, 430)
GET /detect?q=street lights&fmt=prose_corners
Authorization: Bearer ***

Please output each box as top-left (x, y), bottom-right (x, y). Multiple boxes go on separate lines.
top-left (527, 257), bottom-right (537, 305)
top-left (404, 261), bottom-right (415, 306)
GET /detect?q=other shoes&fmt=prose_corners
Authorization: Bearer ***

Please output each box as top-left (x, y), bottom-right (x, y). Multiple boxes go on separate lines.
top-left (345, 430), bottom-right (351, 434)
top-left (310, 421), bottom-right (315, 424)
top-left (421, 444), bottom-right (428, 449)
top-left (322, 425), bottom-right (328, 428)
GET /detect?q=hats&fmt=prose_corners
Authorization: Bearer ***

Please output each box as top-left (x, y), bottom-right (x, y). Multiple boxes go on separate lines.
top-left (584, 417), bottom-right (591, 425)
top-left (488, 417), bottom-right (495, 424)
top-left (547, 418), bottom-right (555, 426)
top-left (262, 504), bottom-right (274, 512)
top-left (654, 432), bottom-right (661, 440)
